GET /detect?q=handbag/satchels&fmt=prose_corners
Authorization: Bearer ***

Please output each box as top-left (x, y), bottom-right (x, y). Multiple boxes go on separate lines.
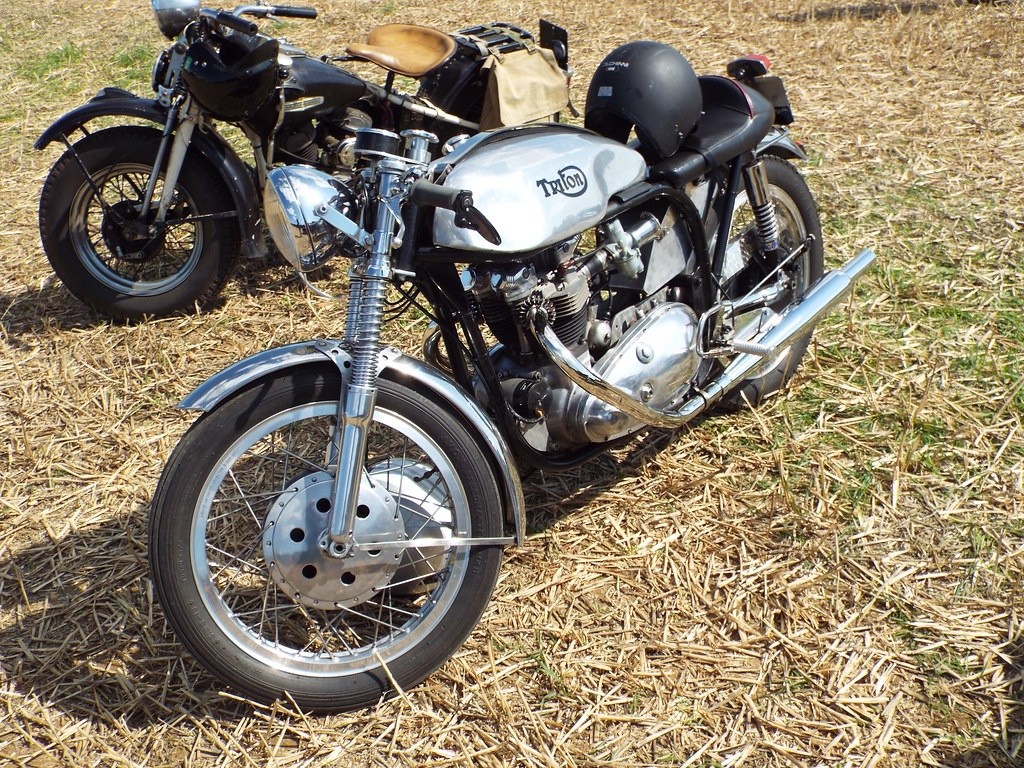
top-left (481, 48), bottom-right (569, 130)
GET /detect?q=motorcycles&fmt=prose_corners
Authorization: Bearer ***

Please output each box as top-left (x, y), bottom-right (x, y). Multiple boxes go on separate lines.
top-left (147, 41), bottom-right (876, 720)
top-left (30, 0), bottom-right (579, 327)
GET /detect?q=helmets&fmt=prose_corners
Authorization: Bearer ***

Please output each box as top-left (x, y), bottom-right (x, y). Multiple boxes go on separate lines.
top-left (585, 43), bottom-right (703, 161)
